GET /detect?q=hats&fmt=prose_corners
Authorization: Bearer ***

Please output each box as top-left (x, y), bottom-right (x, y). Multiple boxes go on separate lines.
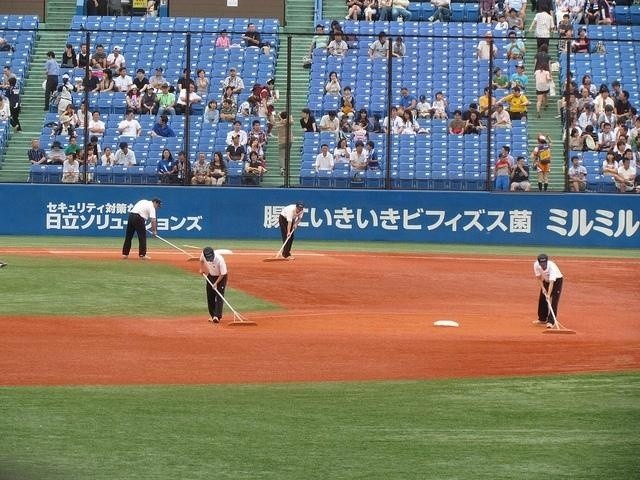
top-left (119, 142), bottom-right (128, 148)
top-left (228, 67), bottom-right (274, 139)
top-left (62, 73), bottom-right (69, 79)
top-left (62, 116), bottom-right (72, 124)
top-left (508, 8), bottom-right (517, 13)
top-left (538, 253), bottom-right (548, 262)
top-left (203, 247), bottom-right (215, 262)
top-left (155, 66), bottom-right (163, 72)
top-left (152, 198), bottom-right (162, 208)
top-left (126, 81), bottom-right (171, 94)
top-left (332, 29), bottom-right (546, 143)
top-left (578, 26), bottom-right (587, 35)
top-left (295, 201), bottom-right (304, 211)
top-left (113, 45), bottom-right (120, 51)
top-left (316, 23), bottom-right (325, 27)
top-left (88, 65), bottom-right (92, 71)
top-left (50, 141), bottom-right (63, 149)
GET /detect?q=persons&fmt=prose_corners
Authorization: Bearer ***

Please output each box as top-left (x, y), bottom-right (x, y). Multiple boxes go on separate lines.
top-left (122, 199), bottom-right (161, 259)
top-left (1, 1), bottom-right (638, 192)
top-left (279, 201), bottom-right (304, 260)
top-left (532, 254), bottom-right (564, 328)
top-left (200, 247), bottom-right (227, 323)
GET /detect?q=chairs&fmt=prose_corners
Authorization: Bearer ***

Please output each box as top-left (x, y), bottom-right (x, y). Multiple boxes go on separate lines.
top-left (0, 12), bottom-right (43, 173)
top-left (298, 21), bottom-right (529, 194)
top-left (609, 5), bottom-right (640, 25)
top-left (27, 0), bottom-right (282, 186)
top-left (408, 1), bottom-right (481, 21)
top-left (557, 25), bottom-right (640, 193)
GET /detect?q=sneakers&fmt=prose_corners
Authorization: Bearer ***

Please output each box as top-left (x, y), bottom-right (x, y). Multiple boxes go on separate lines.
top-left (139, 255), bottom-right (151, 260)
top-left (208, 315), bottom-right (221, 323)
top-left (282, 255), bottom-right (295, 260)
top-left (546, 322), bottom-right (554, 328)
top-left (121, 254), bottom-right (128, 258)
top-left (532, 319), bottom-right (546, 324)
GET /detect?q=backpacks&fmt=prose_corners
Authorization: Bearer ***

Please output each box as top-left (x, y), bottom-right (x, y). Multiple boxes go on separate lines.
top-left (537, 145), bottom-right (551, 163)
